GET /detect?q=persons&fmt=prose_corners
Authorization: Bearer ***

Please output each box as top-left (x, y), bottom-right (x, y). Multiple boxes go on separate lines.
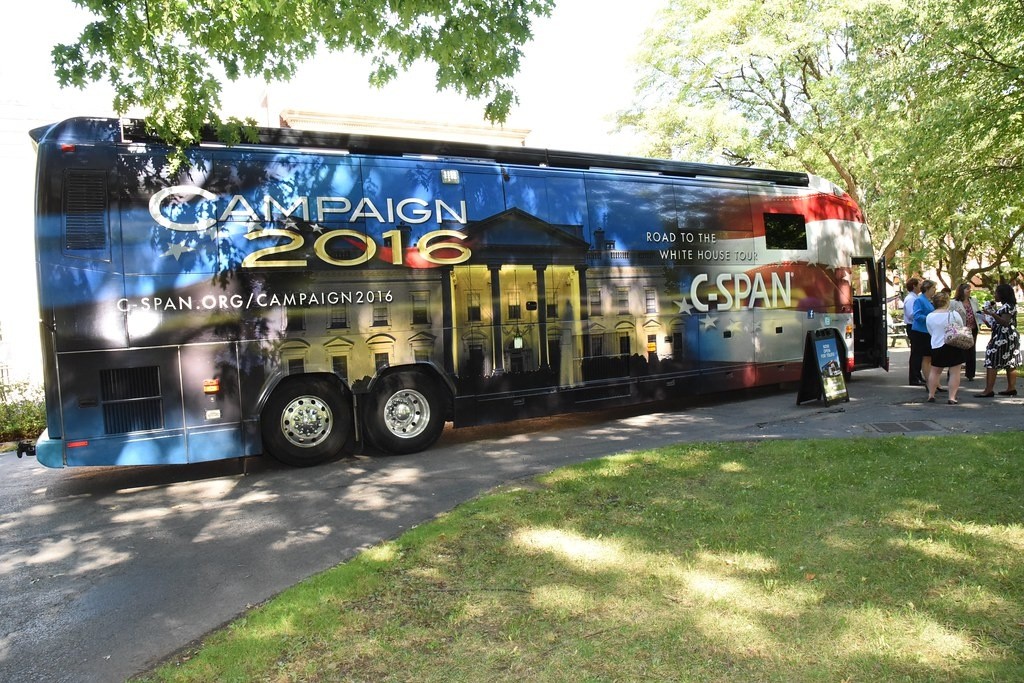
top-left (974, 284), bottom-right (1022, 398)
top-left (969, 297), bottom-right (995, 334)
top-left (947, 283), bottom-right (980, 382)
top-left (908, 280), bottom-right (937, 385)
top-left (904, 278), bottom-right (922, 340)
top-left (926, 292), bottom-right (964, 405)
top-left (941, 288), bottom-right (952, 299)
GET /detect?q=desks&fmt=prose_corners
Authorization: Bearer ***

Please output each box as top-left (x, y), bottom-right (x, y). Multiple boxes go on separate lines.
top-left (887, 323), bottom-right (911, 348)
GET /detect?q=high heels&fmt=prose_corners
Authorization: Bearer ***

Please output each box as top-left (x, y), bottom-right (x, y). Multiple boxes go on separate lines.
top-left (948, 399), bottom-right (957, 404)
top-left (927, 396), bottom-right (935, 402)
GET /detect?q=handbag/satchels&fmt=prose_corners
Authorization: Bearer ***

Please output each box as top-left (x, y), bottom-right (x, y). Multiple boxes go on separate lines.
top-left (944, 310), bottom-right (974, 349)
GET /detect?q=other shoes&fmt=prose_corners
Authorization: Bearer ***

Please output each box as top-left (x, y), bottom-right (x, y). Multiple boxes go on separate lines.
top-left (910, 380), bottom-right (926, 385)
top-left (937, 387), bottom-right (948, 392)
top-left (967, 374), bottom-right (973, 381)
top-left (998, 390), bottom-right (1017, 396)
top-left (974, 391), bottom-right (994, 397)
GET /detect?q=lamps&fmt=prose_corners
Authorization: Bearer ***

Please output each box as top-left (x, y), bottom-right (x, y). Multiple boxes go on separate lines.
top-left (514, 270), bottom-right (523, 349)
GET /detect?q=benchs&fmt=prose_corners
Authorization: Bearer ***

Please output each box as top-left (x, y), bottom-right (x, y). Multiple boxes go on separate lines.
top-left (886, 333), bottom-right (911, 337)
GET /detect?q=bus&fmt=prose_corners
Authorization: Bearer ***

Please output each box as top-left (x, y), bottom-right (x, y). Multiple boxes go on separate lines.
top-left (17, 116), bottom-right (890, 468)
